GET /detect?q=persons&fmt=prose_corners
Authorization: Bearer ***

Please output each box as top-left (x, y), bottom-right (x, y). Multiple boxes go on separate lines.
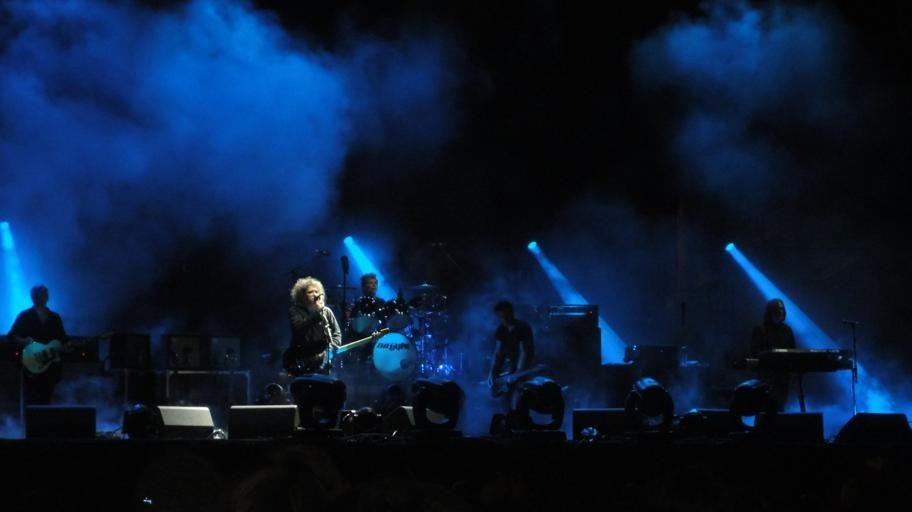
top-left (381, 384), bottom-right (408, 415)
top-left (7, 284), bottom-right (65, 405)
top-left (346, 274), bottom-right (390, 359)
top-left (488, 300), bottom-right (543, 408)
top-left (289, 274), bottom-right (342, 376)
top-left (740, 300), bottom-right (796, 411)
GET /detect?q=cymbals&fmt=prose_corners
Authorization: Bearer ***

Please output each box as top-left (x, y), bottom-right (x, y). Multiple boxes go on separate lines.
top-left (404, 285), bottom-right (444, 291)
top-left (325, 286), bottom-right (357, 290)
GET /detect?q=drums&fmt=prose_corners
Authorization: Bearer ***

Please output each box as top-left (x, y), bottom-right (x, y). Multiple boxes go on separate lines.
top-left (382, 299), bottom-right (412, 332)
top-left (368, 332), bottom-right (417, 382)
top-left (348, 297), bottom-right (386, 334)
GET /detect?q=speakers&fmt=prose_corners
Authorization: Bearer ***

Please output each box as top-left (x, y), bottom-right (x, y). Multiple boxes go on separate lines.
top-left (155, 405), bottom-right (214, 439)
top-left (572, 408), bottom-right (626, 444)
top-left (24, 404), bottom-right (96, 439)
top-left (700, 409), bottom-right (732, 433)
top-left (382, 406), bottom-right (450, 430)
top-left (227, 404), bottom-right (300, 439)
top-left (754, 411), bottom-right (824, 440)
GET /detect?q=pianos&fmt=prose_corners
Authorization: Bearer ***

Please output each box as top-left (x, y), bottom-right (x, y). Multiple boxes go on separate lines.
top-left (756, 349), bottom-right (852, 370)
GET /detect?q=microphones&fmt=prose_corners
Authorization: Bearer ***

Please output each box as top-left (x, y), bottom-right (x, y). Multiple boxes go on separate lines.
top-left (340, 256), bottom-right (350, 274)
top-left (314, 293), bottom-right (323, 301)
top-left (841, 317), bottom-right (859, 326)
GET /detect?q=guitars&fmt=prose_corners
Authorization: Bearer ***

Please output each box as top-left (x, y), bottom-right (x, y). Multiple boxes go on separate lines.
top-left (282, 328), bottom-right (389, 377)
top-left (22, 340), bottom-right (92, 375)
top-left (490, 363), bottom-right (546, 397)
